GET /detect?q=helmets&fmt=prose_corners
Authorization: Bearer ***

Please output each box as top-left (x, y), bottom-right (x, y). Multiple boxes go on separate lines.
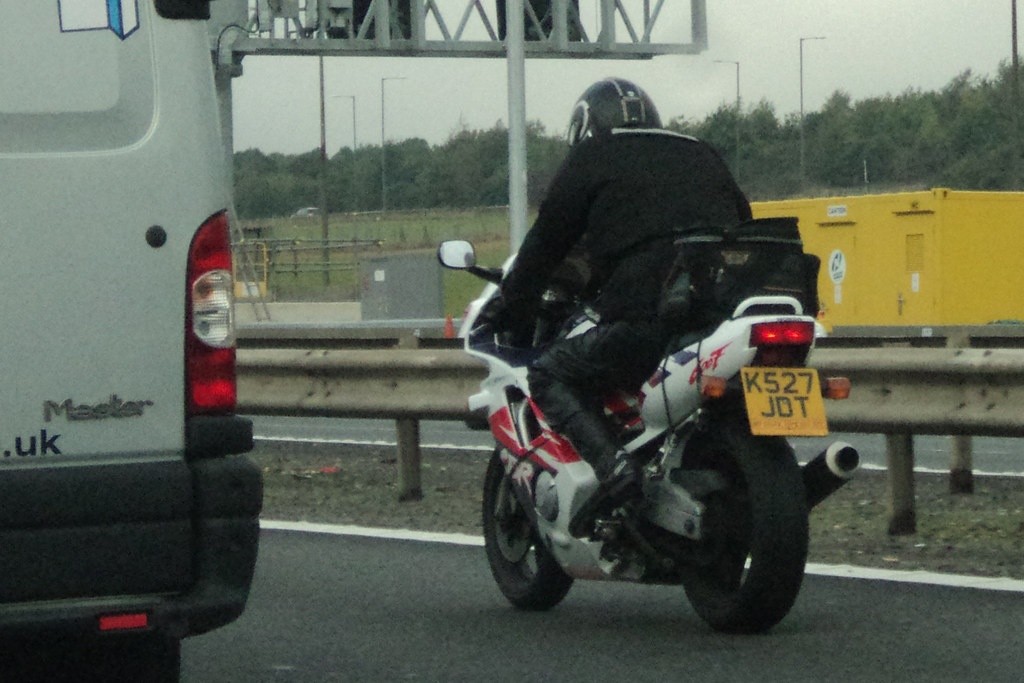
top-left (565, 76), bottom-right (664, 147)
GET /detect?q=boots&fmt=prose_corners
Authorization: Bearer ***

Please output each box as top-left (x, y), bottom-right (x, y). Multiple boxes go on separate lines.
top-left (567, 410), bottom-right (644, 540)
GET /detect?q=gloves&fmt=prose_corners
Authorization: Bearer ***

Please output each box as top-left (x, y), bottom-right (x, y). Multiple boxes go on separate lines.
top-left (479, 296), bottom-right (528, 331)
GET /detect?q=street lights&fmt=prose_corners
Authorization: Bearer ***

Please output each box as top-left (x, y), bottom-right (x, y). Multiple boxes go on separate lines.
top-left (714, 58), bottom-right (742, 193)
top-left (332, 93), bottom-right (360, 210)
top-left (796, 36), bottom-right (825, 197)
top-left (385, 75), bottom-right (407, 215)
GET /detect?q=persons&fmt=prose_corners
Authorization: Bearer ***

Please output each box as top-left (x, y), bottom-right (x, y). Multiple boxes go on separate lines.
top-left (466, 77), bottom-right (755, 537)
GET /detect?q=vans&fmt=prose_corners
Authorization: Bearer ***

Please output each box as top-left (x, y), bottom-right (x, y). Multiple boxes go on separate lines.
top-left (0, 0), bottom-right (269, 683)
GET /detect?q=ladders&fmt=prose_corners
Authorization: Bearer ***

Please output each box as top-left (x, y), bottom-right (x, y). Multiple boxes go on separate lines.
top-left (230, 205), bottom-right (273, 322)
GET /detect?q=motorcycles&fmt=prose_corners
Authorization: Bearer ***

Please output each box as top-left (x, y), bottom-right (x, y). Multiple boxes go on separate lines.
top-left (437, 212), bottom-right (861, 638)
